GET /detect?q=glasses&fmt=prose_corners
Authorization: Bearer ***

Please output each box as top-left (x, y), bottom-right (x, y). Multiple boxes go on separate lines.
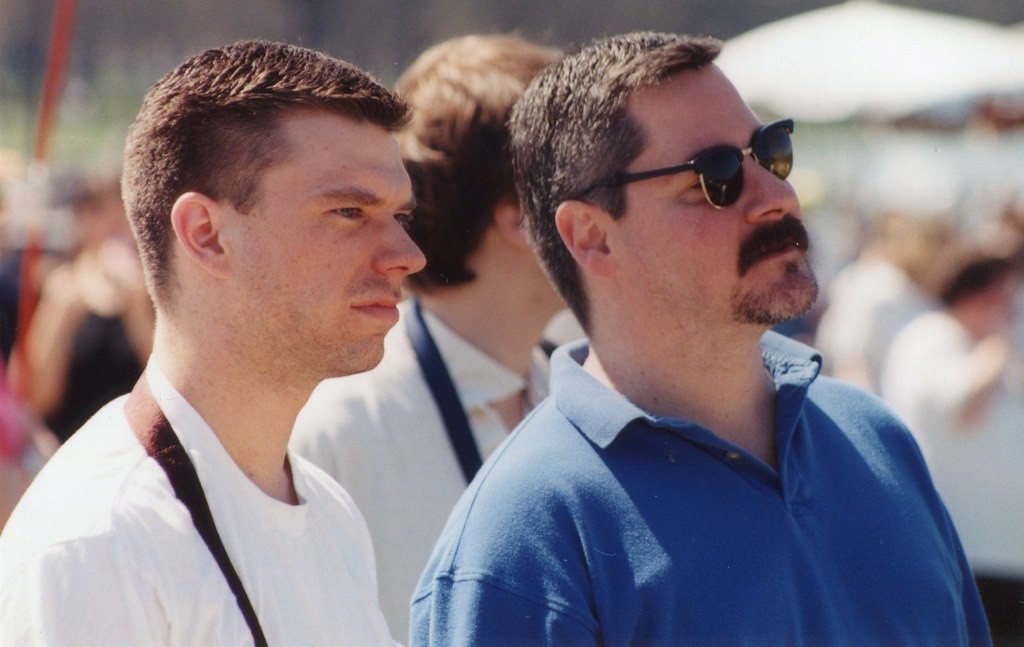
top-left (575, 119), bottom-right (794, 210)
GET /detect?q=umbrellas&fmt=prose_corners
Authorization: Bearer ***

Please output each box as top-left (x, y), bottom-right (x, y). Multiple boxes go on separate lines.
top-left (708, 0), bottom-right (1023, 211)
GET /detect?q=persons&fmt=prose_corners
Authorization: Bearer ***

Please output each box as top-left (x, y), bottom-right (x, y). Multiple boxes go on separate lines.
top-left (408, 30), bottom-right (993, 647)
top-left (812, 197), bottom-right (1024, 647)
top-left (0, 39), bottom-right (426, 647)
top-left (0, 160), bottom-right (155, 447)
top-left (288, 33), bottom-right (569, 647)
